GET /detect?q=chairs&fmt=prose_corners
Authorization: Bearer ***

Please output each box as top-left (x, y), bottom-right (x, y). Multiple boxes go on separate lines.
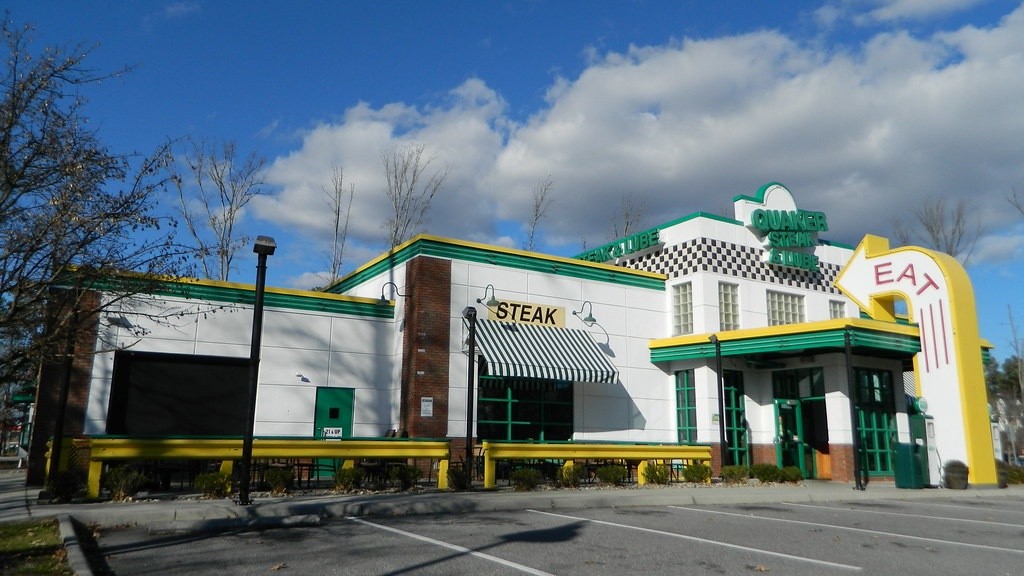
top-left (396, 428), bottom-right (407, 438)
top-left (129, 457), bottom-right (405, 493)
top-left (385, 429), bottom-right (395, 437)
top-left (428, 447), bottom-right (690, 485)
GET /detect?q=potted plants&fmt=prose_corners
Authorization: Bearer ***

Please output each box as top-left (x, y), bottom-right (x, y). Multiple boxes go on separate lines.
top-left (943, 460), bottom-right (969, 489)
top-left (996, 460), bottom-right (1008, 489)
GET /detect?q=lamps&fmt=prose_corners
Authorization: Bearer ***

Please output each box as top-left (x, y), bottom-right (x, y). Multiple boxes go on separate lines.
top-left (573, 301), bottom-right (596, 322)
top-left (298, 375), bottom-right (310, 383)
top-left (377, 282), bottom-right (411, 305)
top-left (476, 284), bottom-right (500, 306)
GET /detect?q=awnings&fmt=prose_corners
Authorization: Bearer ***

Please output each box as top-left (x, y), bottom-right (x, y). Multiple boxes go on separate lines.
top-left (462, 317), bottom-right (620, 385)
